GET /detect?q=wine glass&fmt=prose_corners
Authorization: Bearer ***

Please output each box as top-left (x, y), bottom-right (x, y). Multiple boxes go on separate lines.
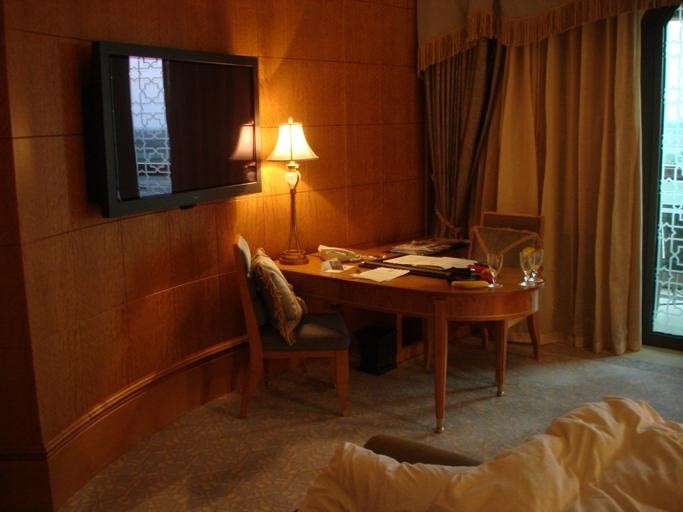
top-left (486, 253), bottom-right (504, 290)
top-left (519, 250), bottom-right (545, 287)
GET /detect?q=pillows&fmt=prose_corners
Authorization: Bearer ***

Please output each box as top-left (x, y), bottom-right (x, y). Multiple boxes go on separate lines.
top-left (250, 245), bottom-right (306, 348)
top-left (469, 227), bottom-right (541, 268)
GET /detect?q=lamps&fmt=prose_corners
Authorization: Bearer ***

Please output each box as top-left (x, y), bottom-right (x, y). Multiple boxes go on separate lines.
top-left (229, 122), bottom-right (255, 182)
top-left (266, 116), bottom-right (319, 266)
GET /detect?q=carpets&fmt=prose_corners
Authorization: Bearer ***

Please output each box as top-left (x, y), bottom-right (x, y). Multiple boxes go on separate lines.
top-left (58, 339), bottom-right (683, 511)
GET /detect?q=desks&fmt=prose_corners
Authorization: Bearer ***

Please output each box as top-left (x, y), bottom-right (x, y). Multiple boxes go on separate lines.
top-left (275, 249), bottom-right (544, 432)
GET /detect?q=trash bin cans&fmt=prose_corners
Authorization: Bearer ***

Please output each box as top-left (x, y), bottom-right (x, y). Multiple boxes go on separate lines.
top-left (352, 324), bottom-right (397, 376)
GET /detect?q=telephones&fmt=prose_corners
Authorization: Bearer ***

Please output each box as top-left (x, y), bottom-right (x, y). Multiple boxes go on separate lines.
top-left (317, 245), bottom-right (356, 263)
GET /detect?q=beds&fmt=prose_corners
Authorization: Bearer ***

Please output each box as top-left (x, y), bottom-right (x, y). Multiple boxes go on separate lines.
top-left (290, 395), bottom-right (683, 511)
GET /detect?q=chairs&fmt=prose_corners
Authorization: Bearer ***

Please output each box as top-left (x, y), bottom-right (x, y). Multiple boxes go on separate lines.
top-left (425, 212), bottom-right (546, 398)
top-left (232, 233), bottom-right (349, 418)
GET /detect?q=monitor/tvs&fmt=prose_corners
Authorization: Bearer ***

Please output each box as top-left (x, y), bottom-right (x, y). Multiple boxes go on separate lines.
top-left (90, 39), bottom-right (262, 217)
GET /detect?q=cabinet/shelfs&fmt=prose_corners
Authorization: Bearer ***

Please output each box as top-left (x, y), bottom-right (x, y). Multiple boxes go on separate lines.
top-left (350, 231), bottom-right (471, 367)
